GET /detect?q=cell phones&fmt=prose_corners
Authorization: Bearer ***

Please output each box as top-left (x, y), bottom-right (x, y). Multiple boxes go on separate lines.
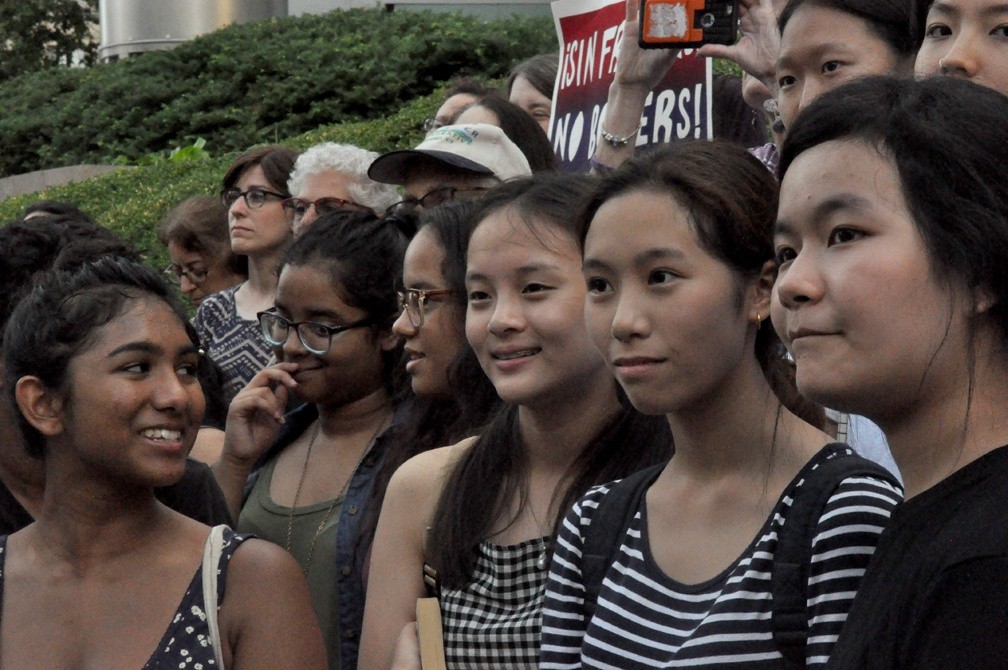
top-left (638, 0), bottom-right (739, 50)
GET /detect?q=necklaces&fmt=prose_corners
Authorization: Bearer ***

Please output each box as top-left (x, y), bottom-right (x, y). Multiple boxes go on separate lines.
top-left (528, 500), bottom-right (548, 570)
top-left (288, 411), bottom-right (389, 576)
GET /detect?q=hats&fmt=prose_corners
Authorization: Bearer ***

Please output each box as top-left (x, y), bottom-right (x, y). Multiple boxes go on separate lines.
top-left (367, 124), bottom-right (532, 183)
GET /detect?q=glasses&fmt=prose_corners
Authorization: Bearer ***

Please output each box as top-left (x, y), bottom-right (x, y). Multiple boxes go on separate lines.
top-left (257, 307), bottom-right (395, 355)
top-left (397, 288), bottom-right (458, 328)
top-left (424, 119), bottom-right (445, 131)
top-left (386, 187), bottom-right (494, 216)
top-left (164, 262), bottom-right (218, 284)
top-left (280, 198), bottom-right (373, 215)
top-left (220, 187), bottom-right (290, 209)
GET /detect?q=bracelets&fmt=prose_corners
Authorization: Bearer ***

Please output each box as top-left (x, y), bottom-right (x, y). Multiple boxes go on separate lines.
top-left (600, 122), bottom-right (642, 145)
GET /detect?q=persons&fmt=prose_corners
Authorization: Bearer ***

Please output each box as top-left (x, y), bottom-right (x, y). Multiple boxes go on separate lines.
top-left (541, 139), bottom-right (904, 670)
top-left (358, 169), bottom-right (608, 670)
top-left (0, 0), bottom-right (1008, 670)
top-left (770, 72), bottom-right (1008, 670)
